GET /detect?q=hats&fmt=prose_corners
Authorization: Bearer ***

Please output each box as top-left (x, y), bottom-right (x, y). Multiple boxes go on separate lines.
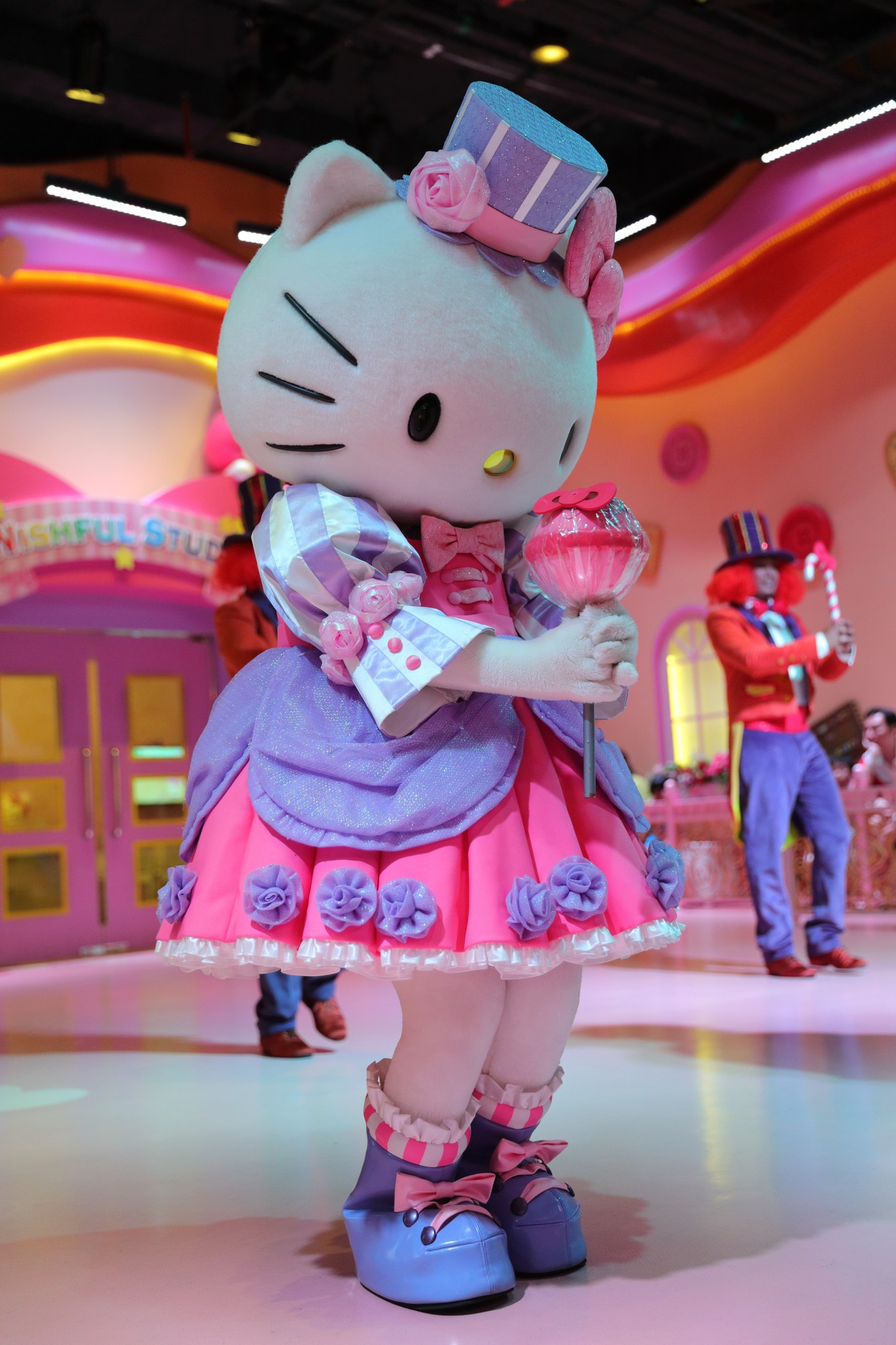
top-left (222, 471), bottom-right (283, 540)
top-left (714, 510), bottom-right (795, 571)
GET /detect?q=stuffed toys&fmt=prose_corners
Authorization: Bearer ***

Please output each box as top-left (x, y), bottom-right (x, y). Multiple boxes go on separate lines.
top-left (156, 79), bottom-right (683, 1308)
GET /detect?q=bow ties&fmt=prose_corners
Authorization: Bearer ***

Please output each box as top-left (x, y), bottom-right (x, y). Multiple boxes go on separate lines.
top-left (752, 598), bottom-right (792, 620)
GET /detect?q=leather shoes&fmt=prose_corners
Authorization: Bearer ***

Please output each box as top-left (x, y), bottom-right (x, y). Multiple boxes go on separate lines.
top-left (261, 1030), bottom-right (313, 1058)
top-left (311, 997), bottom-right (346, 1040)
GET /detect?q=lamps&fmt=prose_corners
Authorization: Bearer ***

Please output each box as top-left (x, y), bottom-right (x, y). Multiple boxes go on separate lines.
top-left (233, 219), bottom-right (279, 246)
top-left (43, 153), bottom-right (190, 228)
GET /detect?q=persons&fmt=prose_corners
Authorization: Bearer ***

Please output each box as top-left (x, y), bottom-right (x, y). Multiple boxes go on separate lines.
top-left (708, 514), bottom-right (896, 980)
top-left (211, 471), bottom-right (347, 1058)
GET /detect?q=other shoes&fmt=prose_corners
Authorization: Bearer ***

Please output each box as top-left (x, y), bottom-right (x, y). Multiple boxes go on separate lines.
top-left (766, 955), bottom-right (816, 977)
top-left (809, 946), bottom-right (867, 968)
